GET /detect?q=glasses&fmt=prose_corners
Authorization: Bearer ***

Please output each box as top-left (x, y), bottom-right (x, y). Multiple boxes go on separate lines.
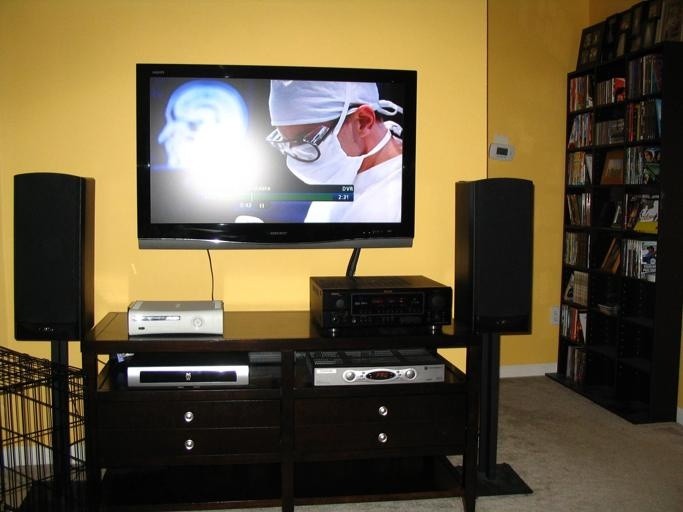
top-left (266, 126), bottom-right (329, 162)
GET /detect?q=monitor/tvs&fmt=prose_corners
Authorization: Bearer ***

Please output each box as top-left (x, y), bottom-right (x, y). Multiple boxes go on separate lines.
top-left (134, 62), bottom-right (418, 251)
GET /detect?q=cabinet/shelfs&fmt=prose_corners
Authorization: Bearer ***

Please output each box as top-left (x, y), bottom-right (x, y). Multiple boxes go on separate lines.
top-left (544, 0), bottom-right (683, 430)
top-left (79, 310), bottom-right (481, 511)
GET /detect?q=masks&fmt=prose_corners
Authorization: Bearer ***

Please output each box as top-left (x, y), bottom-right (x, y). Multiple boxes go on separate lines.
top-left (285, 133), bottom-right (364, 186)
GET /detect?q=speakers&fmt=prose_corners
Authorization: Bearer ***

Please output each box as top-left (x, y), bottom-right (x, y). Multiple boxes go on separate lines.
top-left (14, 172), bottom-right (96, 342)
top-left (454, 177), bottom-right (535, 337)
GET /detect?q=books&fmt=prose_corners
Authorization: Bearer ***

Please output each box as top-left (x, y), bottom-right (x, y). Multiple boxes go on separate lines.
top-left (559, 53), bottom-right (661, 386)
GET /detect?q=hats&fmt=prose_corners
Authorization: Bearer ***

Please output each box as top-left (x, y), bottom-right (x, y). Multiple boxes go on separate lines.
top-left (269, 80), bottom-right (379, 125)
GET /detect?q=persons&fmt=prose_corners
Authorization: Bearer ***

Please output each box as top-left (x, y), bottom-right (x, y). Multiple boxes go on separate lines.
top-left (266, 77), bottom-right (402, 224)
